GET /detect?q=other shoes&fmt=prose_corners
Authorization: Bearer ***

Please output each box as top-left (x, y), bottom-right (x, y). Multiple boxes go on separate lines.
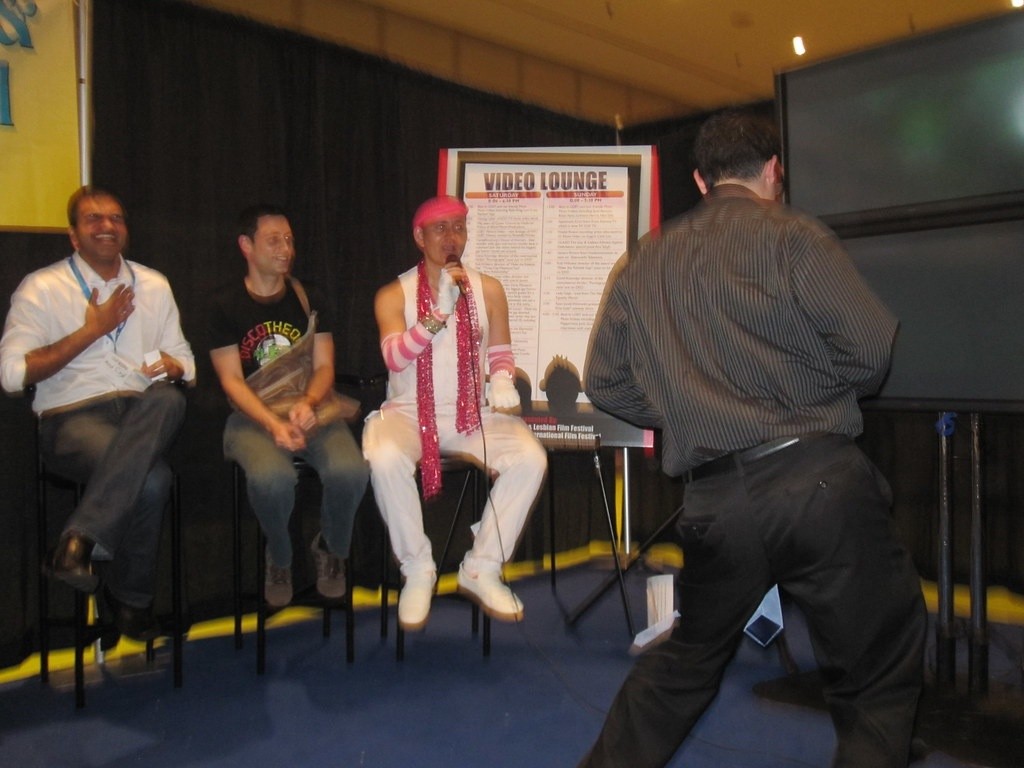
top-left (456, 558), bottom-right (525, 622)
top-left (397, 566), bottom-right (438, 630)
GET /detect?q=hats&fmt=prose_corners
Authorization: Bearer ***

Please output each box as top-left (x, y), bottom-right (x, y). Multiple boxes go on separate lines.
top-left (412, 195), bottom-right (468, 228)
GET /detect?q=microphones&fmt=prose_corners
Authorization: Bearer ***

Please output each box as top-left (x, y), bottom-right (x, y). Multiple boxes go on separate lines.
top-left (445, 254), bottom-right (466, 296)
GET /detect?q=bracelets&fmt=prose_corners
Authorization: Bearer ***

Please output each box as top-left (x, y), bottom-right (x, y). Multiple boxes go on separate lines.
top-left (422, 313), bottom-right (443, 336)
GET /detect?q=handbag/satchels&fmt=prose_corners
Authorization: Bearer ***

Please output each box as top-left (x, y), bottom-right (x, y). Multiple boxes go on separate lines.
top-left (226, 312), bottom-right (360, 429)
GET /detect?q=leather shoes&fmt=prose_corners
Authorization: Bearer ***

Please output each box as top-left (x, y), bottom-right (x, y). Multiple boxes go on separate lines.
top-left (97, 581), bottom-right (161, 641)
top-left (44, 531), bottom-right (99, 594)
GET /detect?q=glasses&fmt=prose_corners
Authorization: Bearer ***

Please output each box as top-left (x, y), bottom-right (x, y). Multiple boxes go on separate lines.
top-left (768, 151), bottom-right (785, 197)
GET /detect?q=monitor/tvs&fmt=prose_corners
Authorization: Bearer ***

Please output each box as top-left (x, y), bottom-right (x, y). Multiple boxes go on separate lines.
top-left (774, 9), bottom-right (1024, 414)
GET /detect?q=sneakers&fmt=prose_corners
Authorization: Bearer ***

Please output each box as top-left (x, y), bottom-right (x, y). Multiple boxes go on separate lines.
top-left (311, 532), bottom-right (347, 598)
top-left (264, 544), bottom-right (293, 607)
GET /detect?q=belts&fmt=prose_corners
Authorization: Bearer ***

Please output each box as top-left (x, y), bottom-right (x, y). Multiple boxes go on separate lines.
top-left (682, 433), bottom-right (799, 483)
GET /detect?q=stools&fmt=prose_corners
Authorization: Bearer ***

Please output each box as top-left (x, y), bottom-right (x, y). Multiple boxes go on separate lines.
top-left (369, 372), bottom-right (493, 667)
top-left (232, 372), bottom-right (361, 676)
top-left (29, 384), bottom-right (182, 708)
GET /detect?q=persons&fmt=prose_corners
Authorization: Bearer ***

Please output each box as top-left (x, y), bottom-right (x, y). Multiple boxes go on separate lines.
top-left (207, 202), bottom-right (371, 607)
top-left (0, 185), bottom-right (196, 641)
top-left (580, 112), bottom-right (929, 768)
top-left (362, 196), bottom-right (548, 631)
